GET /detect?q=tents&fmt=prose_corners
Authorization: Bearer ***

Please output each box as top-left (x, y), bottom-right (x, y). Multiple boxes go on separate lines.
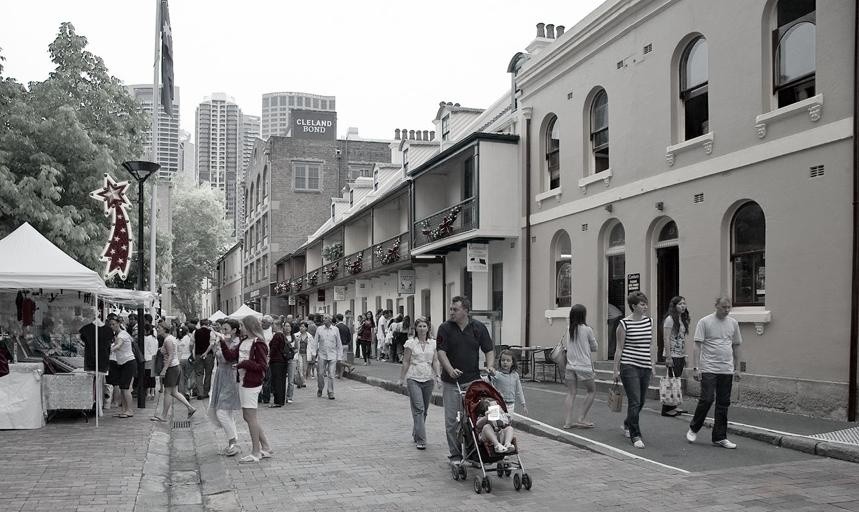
top-left (209, 310), bottom-right (228, 321)
top-left (227, 304), bottom-right (263, 320)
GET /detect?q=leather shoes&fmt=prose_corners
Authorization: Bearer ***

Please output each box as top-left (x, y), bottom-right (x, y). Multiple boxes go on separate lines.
top-left (197, 395), bottom-right (202, 400)
top-left (678, 409), bottom-right (689, 413)
top-left (662, 412), bottom-right (681, 417)
top-left (204, 395), bottom-right (209, 398)
top-left (417, 444), bottom-right (426, 450)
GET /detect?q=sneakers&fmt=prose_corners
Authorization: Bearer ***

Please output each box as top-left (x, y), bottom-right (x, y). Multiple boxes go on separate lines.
top-left (715, 438), bottom-right (737, 449)
top-left (686, 428), bottom-right (698, 442)
top-left (495, 443), bottom-right (508, 453)
top-left (505, 444), bottom-right (515, 453)
top-left (624, 429), bottom-right (631, 438)
top-left (317, 389), bottom-right (323, 397)
top-left (634, 440), bottom-right (646, 450)
top-left (328, 392), bottom-right (335, 399)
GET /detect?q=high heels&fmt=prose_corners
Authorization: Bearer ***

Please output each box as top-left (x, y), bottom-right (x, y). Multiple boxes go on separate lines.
top-left (146, 394), bottom-right (151, 401)
top-left (151, 394), bottom-right (155, 400)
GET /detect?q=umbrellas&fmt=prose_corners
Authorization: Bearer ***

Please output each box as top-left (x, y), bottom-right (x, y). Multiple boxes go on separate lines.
top-left (0, 222), bottom-right (99, 425)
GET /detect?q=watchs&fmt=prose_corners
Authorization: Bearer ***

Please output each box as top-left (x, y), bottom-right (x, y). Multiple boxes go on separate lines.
top-left (436, 375), bottom-right (441, 378)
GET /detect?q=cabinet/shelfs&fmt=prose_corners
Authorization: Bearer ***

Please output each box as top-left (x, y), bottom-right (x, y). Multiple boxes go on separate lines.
top-left (1, 363), bottom-right (45, 431)
top-left (41, 373), bottom-right (95, 422)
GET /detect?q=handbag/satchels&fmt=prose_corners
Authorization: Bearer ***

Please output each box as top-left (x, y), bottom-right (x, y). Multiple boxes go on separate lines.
top-left (150, 348), bottom-right (165, 377)
top-left (607, 378), bottom-right (624, 413)
top-left (548, 327), bottom-right (568, 367)
top-left (659, 367), bottom-right (684, 406)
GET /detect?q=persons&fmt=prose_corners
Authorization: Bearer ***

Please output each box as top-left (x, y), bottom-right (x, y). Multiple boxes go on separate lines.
top-left (476, 398), bottom-right (515, 452)
top-left (399, 319), bottom-right (441, 451)
top-left (662, 295), bottom-right (690, 416)
top-left (356, 312), bottom-right (410, 362)
top-left (435, 297), bottom-right (495, 467)
top-left (276, 314), bottom-right (353, 397)
top-left (81, 309), bottom-right (195, 422)
top-left (686, 298), bottom-right (743, 450)
top-left (607, 292), bottom-right (654, 448)
top-left (203, 321), bottom-right (243, 455)
top-left (211, 316), bottom-right (273, 462)
top-left (489, 351), bottom-right (527, 426)
top-left (565, 305), bottom-right (599, 428)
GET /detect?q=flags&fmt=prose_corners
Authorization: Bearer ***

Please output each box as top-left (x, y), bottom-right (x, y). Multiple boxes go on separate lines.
top-left (160, 0), bottom-right (176, 117)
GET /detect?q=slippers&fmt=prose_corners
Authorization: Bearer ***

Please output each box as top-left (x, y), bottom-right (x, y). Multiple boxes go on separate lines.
top-left (187, 408), bottom-right (197, 419)
top-left (239, 455), bottom-right (259, 464)
top-left (222, 445), bottom-right (242, 457)
top-left (573, 422), bottom-right (596, 428)
top-left (118, 413), bottom-right (133, 418)
top-left (262, 450), bottom-right (275, 458)
top-left (562, 425), bottom-right (575, 429)
top-left (150, 416), bottom-right (168, 422)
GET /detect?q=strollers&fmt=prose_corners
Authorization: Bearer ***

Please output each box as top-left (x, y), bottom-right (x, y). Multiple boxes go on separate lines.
top-left (445, 367), bottom-right (535, 497)
